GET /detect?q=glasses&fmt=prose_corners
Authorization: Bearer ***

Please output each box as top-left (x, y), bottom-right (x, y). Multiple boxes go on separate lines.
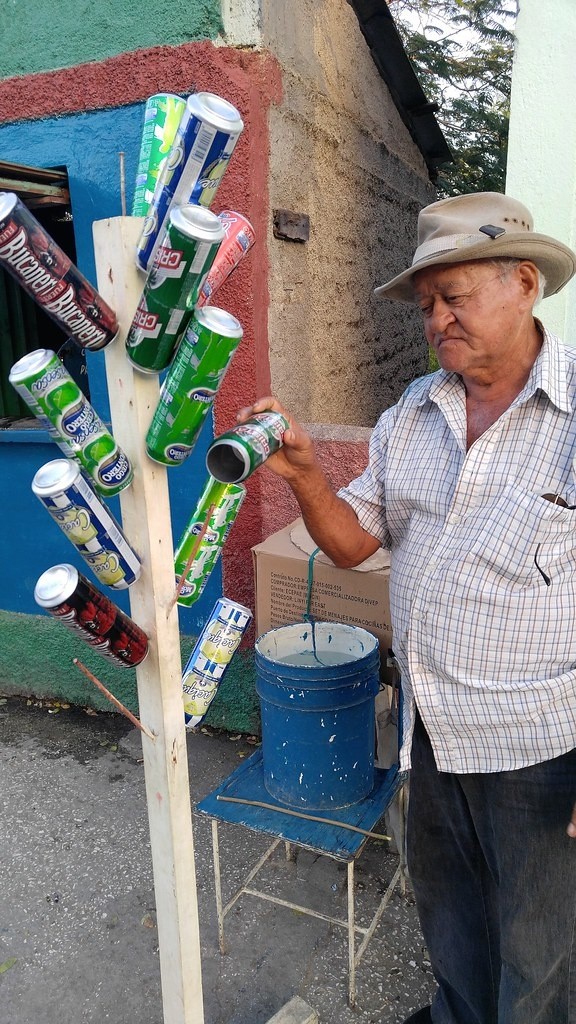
top-left (530, 493), bottom-right (576, 586)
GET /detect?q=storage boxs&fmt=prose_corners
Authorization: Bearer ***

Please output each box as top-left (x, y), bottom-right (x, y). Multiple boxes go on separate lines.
top-left (250, 516), bottom-right (394, 653)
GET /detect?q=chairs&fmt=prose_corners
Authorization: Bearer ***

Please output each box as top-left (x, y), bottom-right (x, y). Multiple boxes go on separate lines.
top-left (195, 665), bottom-right (409, 1007)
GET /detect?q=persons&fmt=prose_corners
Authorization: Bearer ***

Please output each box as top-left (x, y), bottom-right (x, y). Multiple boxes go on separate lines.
top-left (237, 192), bottom-right (576, 1024)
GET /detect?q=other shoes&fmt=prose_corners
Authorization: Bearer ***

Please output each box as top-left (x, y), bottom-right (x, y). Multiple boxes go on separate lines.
top-left (403, 1004), bottom-right (433, 1024)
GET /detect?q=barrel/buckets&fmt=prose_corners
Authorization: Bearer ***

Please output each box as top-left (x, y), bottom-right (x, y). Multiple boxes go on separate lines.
top-left (254, 622), bottom-right (384, 811)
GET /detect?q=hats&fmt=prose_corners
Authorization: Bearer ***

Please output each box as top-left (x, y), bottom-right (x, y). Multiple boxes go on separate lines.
top-left (374, 192), bottom-right (576, 304)
